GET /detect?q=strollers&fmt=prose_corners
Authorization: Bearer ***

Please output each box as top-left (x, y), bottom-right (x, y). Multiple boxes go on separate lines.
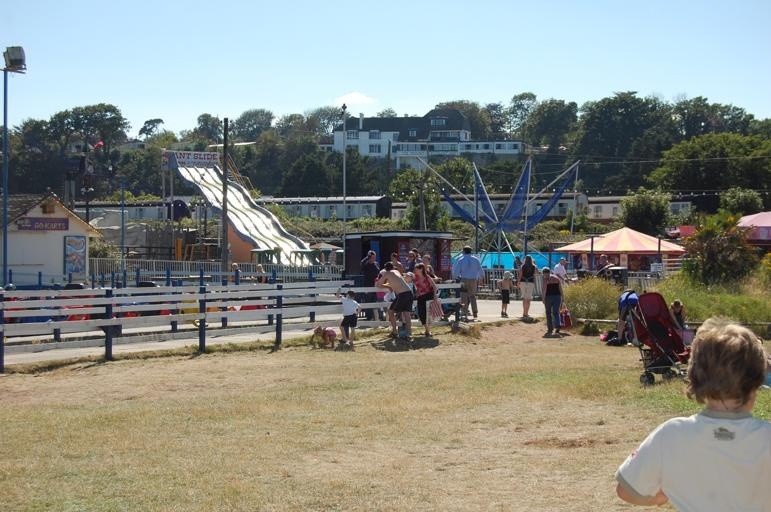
top-left (612, 286), bottom-right (644, 348)
top-left (625, 290), bottom-right (696, 388)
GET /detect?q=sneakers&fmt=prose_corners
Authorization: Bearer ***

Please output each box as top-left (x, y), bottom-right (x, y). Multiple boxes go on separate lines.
top-left (387, 331), bottom-right (410, 342)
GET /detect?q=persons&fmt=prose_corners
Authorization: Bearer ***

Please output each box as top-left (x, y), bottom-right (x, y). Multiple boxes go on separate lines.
top-left (497, 271), bottom-right (519, 317)
top-left (541, 267), bottom-right (567, 336)
top-left (256, 264), bottom-right (269, 309)
top-left (310, 326), bottom-right (336, 349)
top-left (336, 287), bottom-right (363, 346)
top-left (554, 257), bottom-right (573, 288)
top-left (453, 246), bottom-right (486, 318)
top-left (617, 289), bottom-right (639, 345)
top-left (617, 314), bottom-right (771, 511)
top-left (250, 268), bottom-right (260, 285)
top-left (517, 255), bottom-right (542, 320)
top-left (596, 254), bottom-right (609, 272)
top-left (669, 299), bottom-right (687, 347)
top-left (515, 256), bottom-right (521, 268)
top-left (360, 247), bottom-right (462, 339)
top-left (532, 260), bottom-right (542, 301)
top-left (231, 262), bottom-right (243, 285)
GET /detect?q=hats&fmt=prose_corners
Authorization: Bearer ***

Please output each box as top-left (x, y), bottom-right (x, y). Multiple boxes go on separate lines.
top-left (671, 297), bottom-right (683, 308)
top-left (560, 256), bottom-right (566, 261)
top-left (503, 270), bottom-right (514, 281)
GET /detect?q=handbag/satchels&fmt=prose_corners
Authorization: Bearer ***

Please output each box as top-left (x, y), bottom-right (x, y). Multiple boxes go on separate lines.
top-left (559, 302), bottom-right (572, 328)
top-left (431, 299), bottom-right (444, 320)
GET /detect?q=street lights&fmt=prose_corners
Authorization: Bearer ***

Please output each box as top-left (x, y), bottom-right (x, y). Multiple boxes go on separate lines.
top-left (2, 44), bottom-right (26, 289)
